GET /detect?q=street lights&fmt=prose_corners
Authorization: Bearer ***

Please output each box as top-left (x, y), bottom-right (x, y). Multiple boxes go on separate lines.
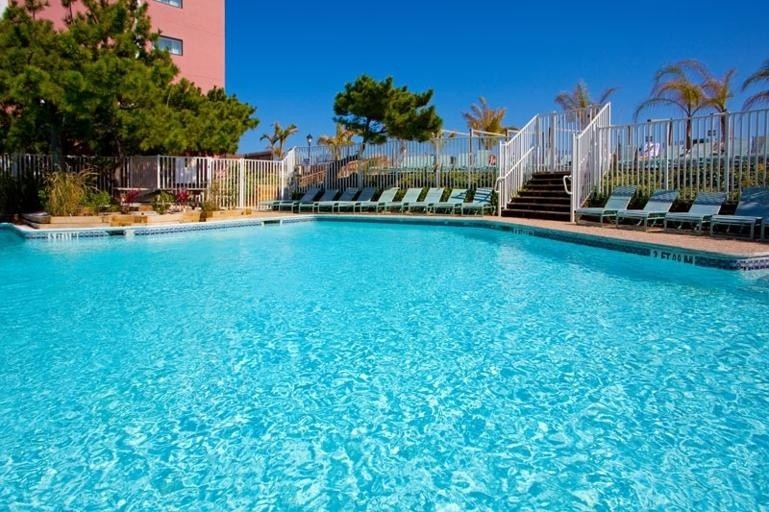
top-left (307, 134), bottom-right (312, 158)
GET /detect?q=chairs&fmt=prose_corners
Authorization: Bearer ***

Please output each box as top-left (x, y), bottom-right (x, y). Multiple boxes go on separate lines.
top-left (572, 185), bottom-right (769, 245)
top-left (258, 185), bottom-right (495, 218)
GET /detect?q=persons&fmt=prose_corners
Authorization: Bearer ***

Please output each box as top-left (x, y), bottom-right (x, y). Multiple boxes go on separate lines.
top-left (642, 135), bottom-right (654, 159)
